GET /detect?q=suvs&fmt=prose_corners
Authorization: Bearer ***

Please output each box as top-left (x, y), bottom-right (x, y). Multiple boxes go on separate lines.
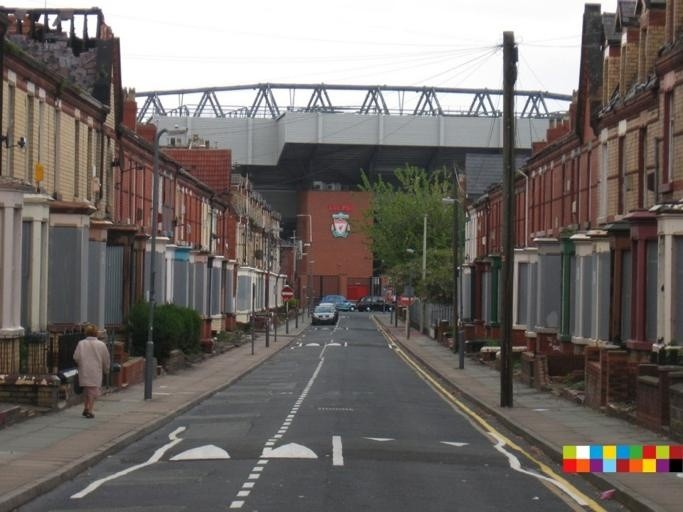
top-left (321, 294), bottom-right (356, 312)
top-left (356, 295), bottom-right (396, 312)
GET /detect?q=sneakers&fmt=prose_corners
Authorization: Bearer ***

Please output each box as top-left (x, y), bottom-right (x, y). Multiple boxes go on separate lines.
top-left (83, 408), bottom-right (96, 419)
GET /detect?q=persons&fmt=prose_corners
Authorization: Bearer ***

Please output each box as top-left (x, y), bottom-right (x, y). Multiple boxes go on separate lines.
top-left (71, 324), bottom-right (111, 420)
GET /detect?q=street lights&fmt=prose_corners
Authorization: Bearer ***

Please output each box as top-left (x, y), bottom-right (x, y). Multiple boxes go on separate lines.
top-left (440, 195), bottom-right (466, 373)
top-left (144, 124), bottom-right (188, 400)
top-left (404, 247), bottom-right (426, 334)
top-left (261, 225), bottom-right (314, 350)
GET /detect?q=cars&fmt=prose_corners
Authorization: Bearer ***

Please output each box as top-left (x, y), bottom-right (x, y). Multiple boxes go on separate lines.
top-left (311, 300), bottom-right (339, 324)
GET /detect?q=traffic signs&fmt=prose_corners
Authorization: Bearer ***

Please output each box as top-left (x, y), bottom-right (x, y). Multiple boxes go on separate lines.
top-left (399, 292), bottom-right (416, 305)
